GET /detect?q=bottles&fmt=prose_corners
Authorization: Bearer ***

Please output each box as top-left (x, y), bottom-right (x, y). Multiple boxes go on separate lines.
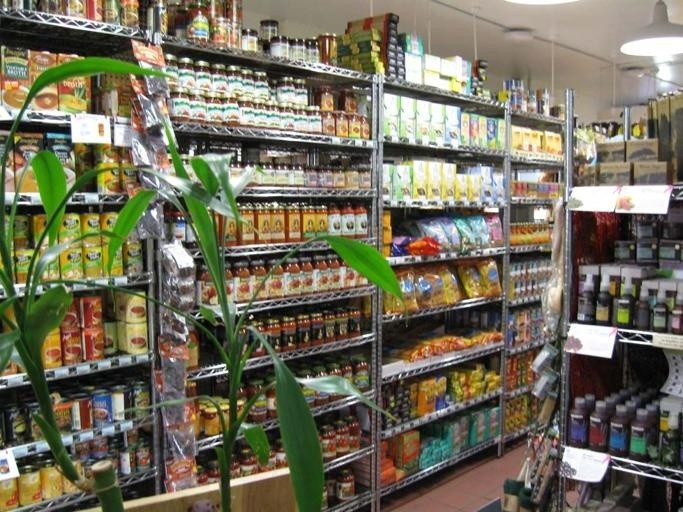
top-left (574, 270), bottom-right (683, 335)
top-left (564, 382), bottom-right (683, 470)
top-left (541, 87), bottom-right (550, 117)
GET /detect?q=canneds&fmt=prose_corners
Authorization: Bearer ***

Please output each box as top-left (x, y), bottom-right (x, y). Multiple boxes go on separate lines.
top-left (165, 0), bottom-right (370, 510)
top-left (0, 211), bottom-right (152, 512)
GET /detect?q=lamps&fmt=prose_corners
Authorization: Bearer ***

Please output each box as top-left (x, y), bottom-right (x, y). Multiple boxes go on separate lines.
top-left (618, 1), bottom-right (682, 58)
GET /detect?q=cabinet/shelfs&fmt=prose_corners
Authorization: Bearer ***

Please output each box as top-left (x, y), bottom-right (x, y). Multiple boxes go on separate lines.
top-left (0, 4), bottom-right (375, 510)
top-left (374, 75), bottom-right (570, 512)
top-left (532, 86), bottom-right (682, 510)
